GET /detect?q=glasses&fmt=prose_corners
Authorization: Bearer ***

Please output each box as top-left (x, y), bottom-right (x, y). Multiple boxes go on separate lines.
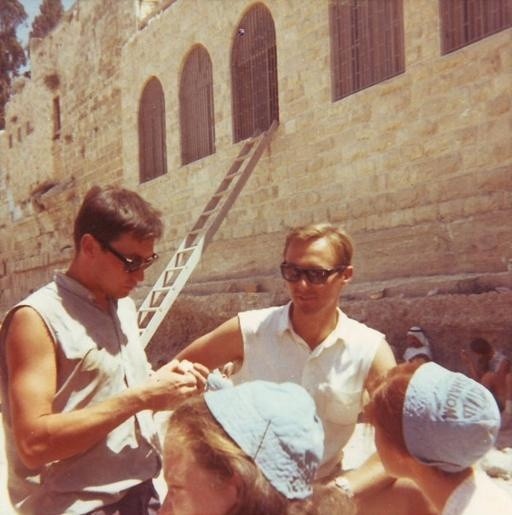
top-left (280, 262), bottom-right (345, 284)
top-left (104, 242), bottom-right (159, 272)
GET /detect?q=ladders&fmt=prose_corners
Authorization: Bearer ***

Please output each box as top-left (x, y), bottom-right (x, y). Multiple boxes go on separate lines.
top-left (136, 119), bottom-right (278, 350)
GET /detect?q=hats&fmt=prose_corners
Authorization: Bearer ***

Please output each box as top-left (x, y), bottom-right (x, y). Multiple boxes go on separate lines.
top-left (202, 381), bottom-right (326, 498)
top-left (403, 362), bottom-right (502, 473)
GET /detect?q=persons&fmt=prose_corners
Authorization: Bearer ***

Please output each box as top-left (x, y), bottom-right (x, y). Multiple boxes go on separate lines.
top-left (402, 327), bottom-right (433, 363)
top-left (156, 378), bottom-right (325, 514)
top-left (1, 182), bottom-right (206, 515)
top-left (462, 337), bottom-right (508, 413)
top-left (371, 358), bottom-right (511, 514)
top-left (168, 223), bottom-right (399, 483)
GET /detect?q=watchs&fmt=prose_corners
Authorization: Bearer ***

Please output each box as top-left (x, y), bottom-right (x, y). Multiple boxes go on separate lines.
top-left (333, 477), bottom-right (354, 499)
